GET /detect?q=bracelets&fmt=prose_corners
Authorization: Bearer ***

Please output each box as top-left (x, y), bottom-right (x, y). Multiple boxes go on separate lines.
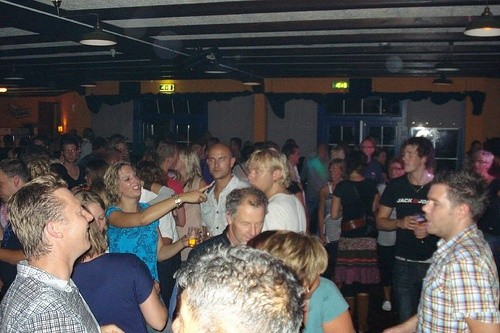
top-left (171, 193), bottom-right (183, 208)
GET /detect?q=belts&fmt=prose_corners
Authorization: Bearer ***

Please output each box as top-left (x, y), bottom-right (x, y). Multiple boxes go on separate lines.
top-left (394, 256), bottom-right (433, 263)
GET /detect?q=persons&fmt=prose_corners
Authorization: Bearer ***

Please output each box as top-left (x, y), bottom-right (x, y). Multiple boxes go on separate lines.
top-left (0, 127), bottom-right (500, 333)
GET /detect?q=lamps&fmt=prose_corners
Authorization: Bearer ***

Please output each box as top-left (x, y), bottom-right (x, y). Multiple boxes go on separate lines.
top-left (432, 56), bottom-right (460, 86)
top-left (463, 0), bottom-right (500, 37)
top-left (52, 0), bottom-right (117, 46)
top-left (3, 65), bottom-right (26, 81)
top-left (241, 69), bottom-right (260, 86)
top-left (79, 70), bottom-right (96, 88)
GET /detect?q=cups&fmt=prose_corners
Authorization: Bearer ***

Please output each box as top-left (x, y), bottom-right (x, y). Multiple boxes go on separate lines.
top-left (187, 226), bottom-right (199, 247)
top-left (415, 217), bottom-right (425, 228)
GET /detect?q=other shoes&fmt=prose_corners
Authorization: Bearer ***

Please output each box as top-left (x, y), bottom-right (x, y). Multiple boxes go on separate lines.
top-left (383, 301), bottom-right (391, 311)
